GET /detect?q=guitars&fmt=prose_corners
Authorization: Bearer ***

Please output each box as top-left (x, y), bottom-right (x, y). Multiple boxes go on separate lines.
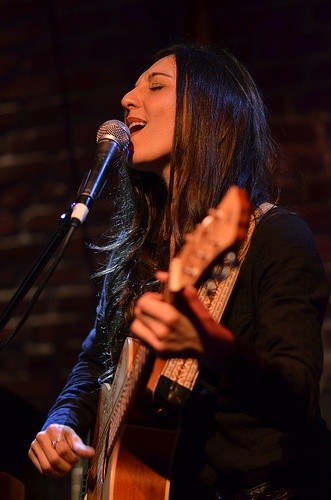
top-left (81, 186), bottom-right (250, 500)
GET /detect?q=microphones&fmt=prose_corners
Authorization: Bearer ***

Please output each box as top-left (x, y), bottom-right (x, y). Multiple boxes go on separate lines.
top-left (69, 120), bottom-right (130, 229)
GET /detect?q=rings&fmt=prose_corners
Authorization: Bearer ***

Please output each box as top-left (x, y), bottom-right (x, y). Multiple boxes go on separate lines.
top-left (51, 437), bottom-right (65, 447)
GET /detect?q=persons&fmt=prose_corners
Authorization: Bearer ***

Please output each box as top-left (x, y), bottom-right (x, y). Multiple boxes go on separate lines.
top-left (26, 42), bottom-right (331, 500)
